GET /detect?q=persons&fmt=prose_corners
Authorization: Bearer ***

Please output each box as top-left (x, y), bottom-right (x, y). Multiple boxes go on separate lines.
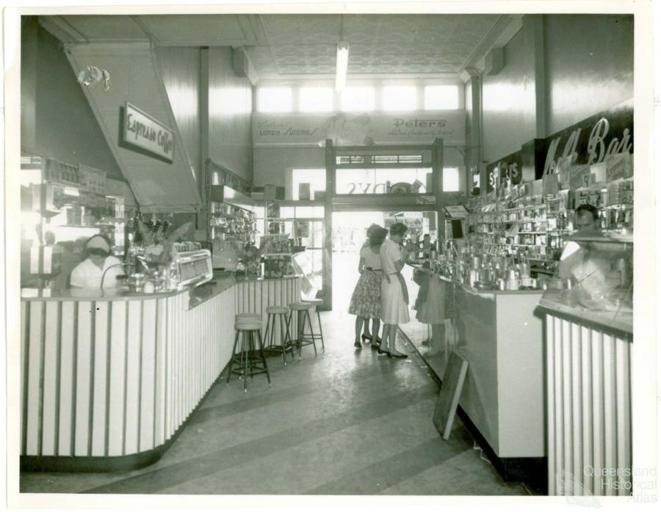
top-left (409, 268), bottom-right (472, 361)
top-left (348, 226), bottom-right (389, 350)
top-left (378, 223), bottom-right (415, 359)
top-left (360, 223), bottom-right (381, 343)
top-left (68, 233), bottom-right (126, 288)
top-left (559, 202), bottom-right (627, 294)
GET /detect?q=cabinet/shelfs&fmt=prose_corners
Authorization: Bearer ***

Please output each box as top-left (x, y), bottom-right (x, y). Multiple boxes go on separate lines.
top-left (469, 177), bottom-right (632, 278)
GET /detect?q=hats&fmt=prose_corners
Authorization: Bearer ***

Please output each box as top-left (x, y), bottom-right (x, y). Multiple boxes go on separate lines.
top-left (86, 233), bottom-right (110, 256)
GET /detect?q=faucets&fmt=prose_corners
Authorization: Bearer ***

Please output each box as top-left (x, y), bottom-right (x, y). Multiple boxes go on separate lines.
top-left (100, 264), bottom-right (128, 290)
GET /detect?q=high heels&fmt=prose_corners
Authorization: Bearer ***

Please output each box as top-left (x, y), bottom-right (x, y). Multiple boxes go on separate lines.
top-left (354, 333), bottom-right (408, 358)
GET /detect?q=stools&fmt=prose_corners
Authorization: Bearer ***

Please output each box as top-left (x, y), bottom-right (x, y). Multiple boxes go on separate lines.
top-left (227, 298), bottom-right (325, 394)
